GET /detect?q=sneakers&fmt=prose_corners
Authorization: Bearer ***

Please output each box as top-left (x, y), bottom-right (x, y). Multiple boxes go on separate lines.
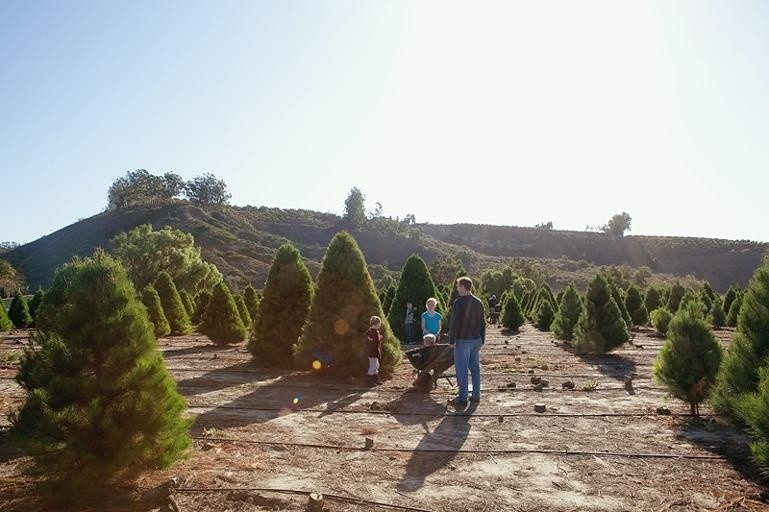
top-left (469, 396), bottom-right (480, 402)
top-left (365, 380), bottom-right (377, 387)
top-left (447, 397), bottom-right (467, 405)
top-left (371, 378), bottom-right (383, 385)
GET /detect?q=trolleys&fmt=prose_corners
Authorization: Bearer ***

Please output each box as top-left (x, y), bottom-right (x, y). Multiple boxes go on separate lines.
top-left (404, 344), bottom-right (456, 393)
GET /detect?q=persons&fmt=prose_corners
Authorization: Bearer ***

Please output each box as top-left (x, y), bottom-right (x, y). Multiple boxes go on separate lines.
top-left (365, 316), bottom-right (383, 387)
top-left (489, 295), bottom-right (498, 318)
top-left (420, 297), bottom-right (443, 344)
top-left (422, 333), bottom-right (436, 348)
top-left (446, 276), bottom-right (486, 405)
top-left (403, 301), bottom-right (418, 345)
